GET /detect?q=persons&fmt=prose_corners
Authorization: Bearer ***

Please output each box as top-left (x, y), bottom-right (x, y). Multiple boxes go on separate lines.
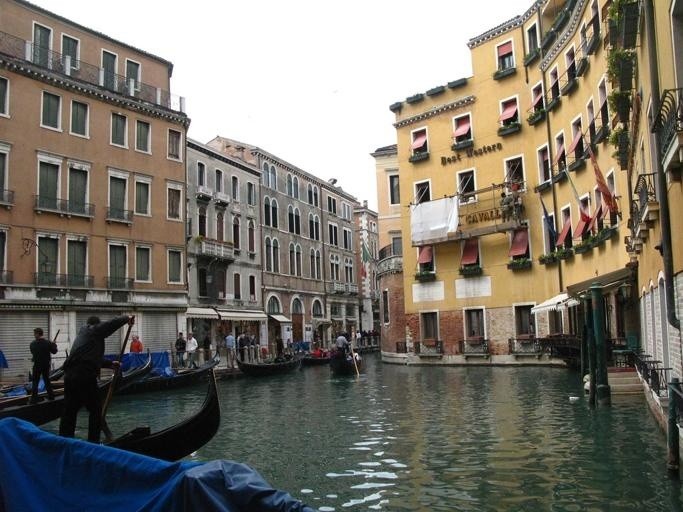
top-left (174, 332), bottom-right (186, 369)
top-left (58, 315), bottom-right (135, 443)
top-left (276, 337), bottom-right (284, 356)
top-left (330, 329), bottom-right (381, 362)
top-left (185, 332), bottom-right (198, 369)
top-left (309, 347), bottom-right (321, 357)
top-left (224, 332), bottom-right (256, 368)
top-left (499, 192), bottom-right (513, 224)
top-left (26, 328), bottom-right (57, 405)
top-left (202, 335), bottom-right (210, 361)
top-left (130, 335), bottom-right (143, 353)
top-left (287, 338), bottom-right (292, 355)
top-left (514, 192), bottom-right (522, 224)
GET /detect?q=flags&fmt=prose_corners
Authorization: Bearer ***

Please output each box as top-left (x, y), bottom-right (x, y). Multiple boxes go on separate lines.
top-left (362, 239), bottom-right (372, 262)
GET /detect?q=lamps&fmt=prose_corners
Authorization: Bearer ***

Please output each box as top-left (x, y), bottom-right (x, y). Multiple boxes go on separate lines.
top-left (617, 279), bottom-right (641, 307)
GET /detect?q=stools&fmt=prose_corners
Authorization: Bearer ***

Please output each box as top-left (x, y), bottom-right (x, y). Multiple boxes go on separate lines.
top-left (611, 350), bottom-right (632, 369)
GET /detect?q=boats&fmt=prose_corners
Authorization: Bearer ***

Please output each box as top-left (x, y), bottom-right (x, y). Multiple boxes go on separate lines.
top-left (236, 353), bottom-right (306, 376)
top-left (331, 348), bottom-right (360, 377)
top-left (1, 352), bottom-right (220, 400)
top-left (301, 349), bottom-right (334, 367)
top-left (0, 345), bottom-right (154, 426)
top-left (101, 367), bottom-right (222, 463)
top-left (0, 417), bottom-right (312, 512)
